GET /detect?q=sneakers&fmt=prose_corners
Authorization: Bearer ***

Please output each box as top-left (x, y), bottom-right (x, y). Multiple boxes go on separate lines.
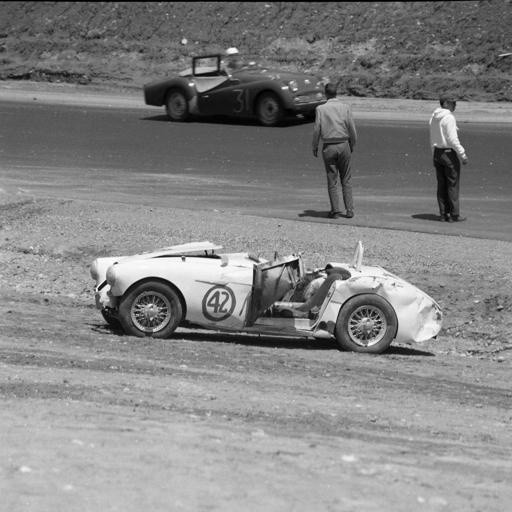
top-left (329, 210), bottom-right (353, 219)
top-left (440, 214), bottom-right (467, 222)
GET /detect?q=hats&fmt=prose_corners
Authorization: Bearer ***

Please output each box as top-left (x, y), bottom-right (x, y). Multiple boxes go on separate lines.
top-left (438, 93), bottom-right (458, 106)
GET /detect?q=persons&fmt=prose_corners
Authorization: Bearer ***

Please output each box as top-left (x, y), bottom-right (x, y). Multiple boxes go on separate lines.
top-left (428, 93), bottom-right (468, 222)
top-left (311, 83), bottom-right (358, 218)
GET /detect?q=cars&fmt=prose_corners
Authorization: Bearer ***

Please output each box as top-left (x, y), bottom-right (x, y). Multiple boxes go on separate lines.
top-left (90, 239), bottom-right (442, 354)
top-left (143, 53), bottom-right (325, 126)
